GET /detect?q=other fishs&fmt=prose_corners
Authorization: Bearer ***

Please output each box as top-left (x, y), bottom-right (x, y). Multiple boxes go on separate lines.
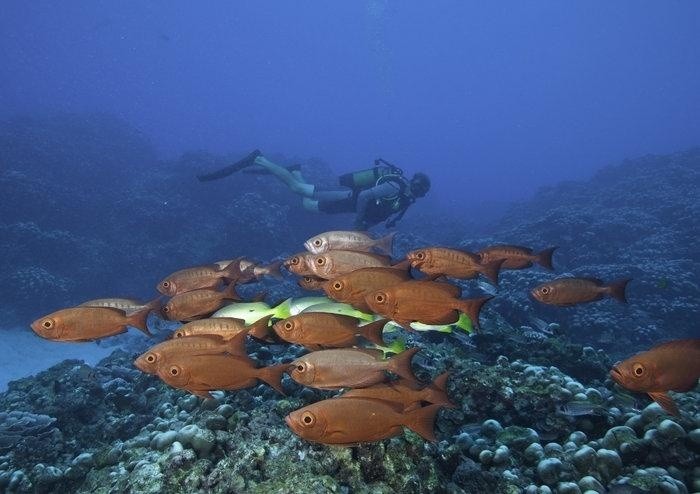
top-left (132, 229), bottom-right (631, 447)
top-left (77, 294), bottom-right (167, 324)
top-left (529, 279), bottom-right (630, 308)
top-left (30, 305), bottom-right (156, 344)
top-left (609, 339), bottom-right (700, 419)
top-left (474, 239), bottom-right (562, 275)
top-left (132, 232), bottom-right (505, 449)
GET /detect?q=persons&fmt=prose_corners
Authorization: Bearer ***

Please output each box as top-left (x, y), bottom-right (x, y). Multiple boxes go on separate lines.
top-left (241, 149), bottom-right (433, 234)
